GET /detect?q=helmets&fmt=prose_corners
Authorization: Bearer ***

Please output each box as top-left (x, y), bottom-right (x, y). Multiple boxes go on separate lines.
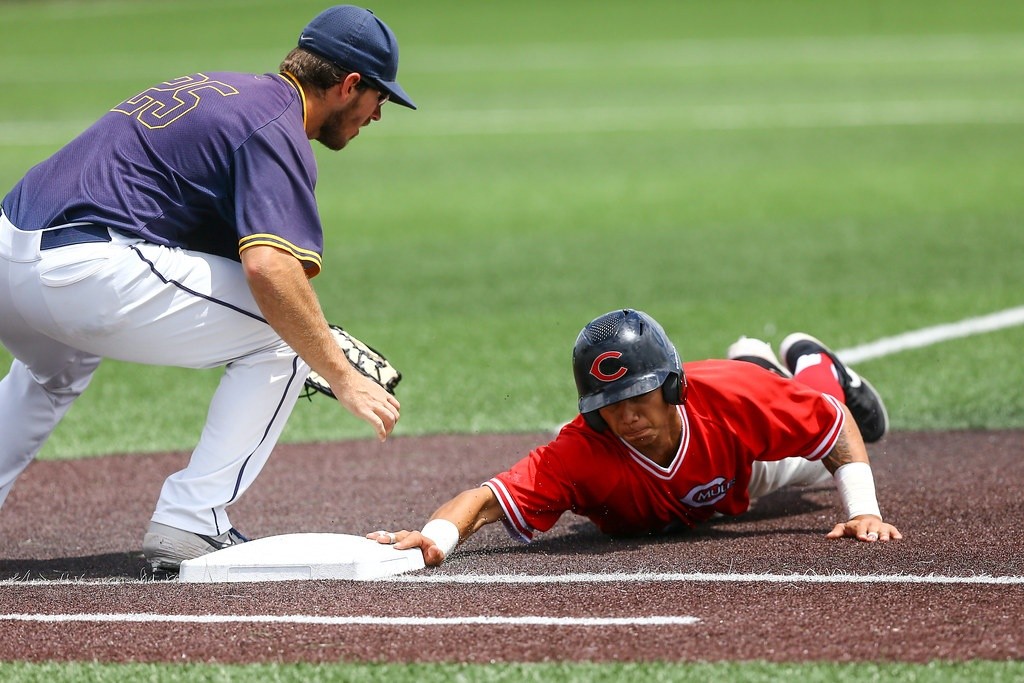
top-left (572, 309), bottom-right (688, 433)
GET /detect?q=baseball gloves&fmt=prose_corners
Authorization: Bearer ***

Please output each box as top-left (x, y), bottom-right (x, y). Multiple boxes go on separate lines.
top-left (296, 323), bottom-right (409, 405)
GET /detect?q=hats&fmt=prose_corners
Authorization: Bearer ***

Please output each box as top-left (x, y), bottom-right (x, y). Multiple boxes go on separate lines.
top-left (298, 4), bottom-right (417, 110)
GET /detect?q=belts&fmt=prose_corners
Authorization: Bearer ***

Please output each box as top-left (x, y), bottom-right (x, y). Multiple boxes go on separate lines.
top-left (40, 224), bottom-right (112, 252)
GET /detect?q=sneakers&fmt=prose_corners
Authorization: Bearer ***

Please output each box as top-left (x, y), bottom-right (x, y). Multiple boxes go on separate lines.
top-left (141, 519), bottom-right (255, 568)
top-left (779, 332), bottom-right (890, 443)
top-left (727, 338), bottom-right (793, 379)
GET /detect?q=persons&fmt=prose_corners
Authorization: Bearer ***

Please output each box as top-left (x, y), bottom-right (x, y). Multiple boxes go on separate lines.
top-left (366, 307), bottom-right (902, 569)
top-left (0, 5), bottom-right (416, 570)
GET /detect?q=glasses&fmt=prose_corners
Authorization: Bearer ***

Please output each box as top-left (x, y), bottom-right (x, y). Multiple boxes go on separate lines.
top-left (334, 61), bottom-right (391, 106)
